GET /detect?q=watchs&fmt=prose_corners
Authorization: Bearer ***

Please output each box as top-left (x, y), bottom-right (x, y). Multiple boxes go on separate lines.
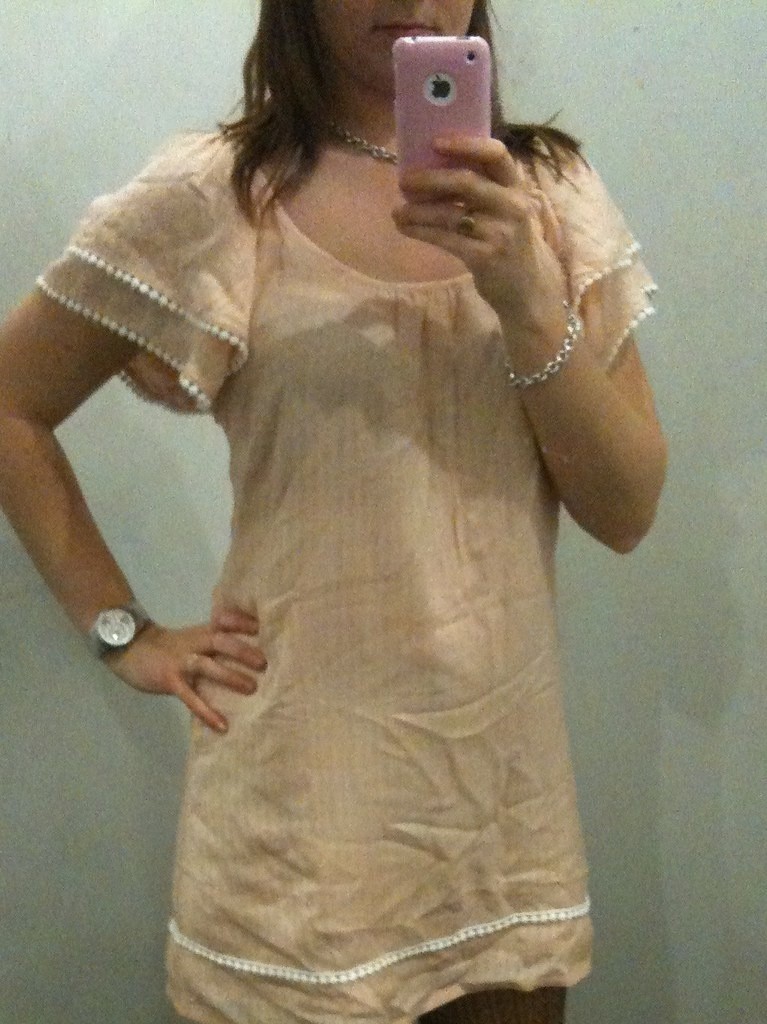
top-left (81, 604), bottom-right (151, 661)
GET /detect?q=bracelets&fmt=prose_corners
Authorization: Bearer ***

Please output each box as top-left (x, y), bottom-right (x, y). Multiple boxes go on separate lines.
top-left (506, 299), bottom-right (583, 389)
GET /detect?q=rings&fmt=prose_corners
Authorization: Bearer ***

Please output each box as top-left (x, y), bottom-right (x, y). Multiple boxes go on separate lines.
top-left (459, 205), bottom-right (479, 237)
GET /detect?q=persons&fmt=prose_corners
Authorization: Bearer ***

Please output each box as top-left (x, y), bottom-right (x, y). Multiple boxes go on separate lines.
top-left (1, 0), bottom-right (665, 1024)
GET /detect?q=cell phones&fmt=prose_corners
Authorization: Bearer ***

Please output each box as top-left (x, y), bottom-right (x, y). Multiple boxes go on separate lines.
top-left (393, 32), bottom-right (492, 205)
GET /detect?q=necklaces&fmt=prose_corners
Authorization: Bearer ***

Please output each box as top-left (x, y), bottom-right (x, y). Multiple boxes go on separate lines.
top-left (324, 111), bottom-right (399, 167)
